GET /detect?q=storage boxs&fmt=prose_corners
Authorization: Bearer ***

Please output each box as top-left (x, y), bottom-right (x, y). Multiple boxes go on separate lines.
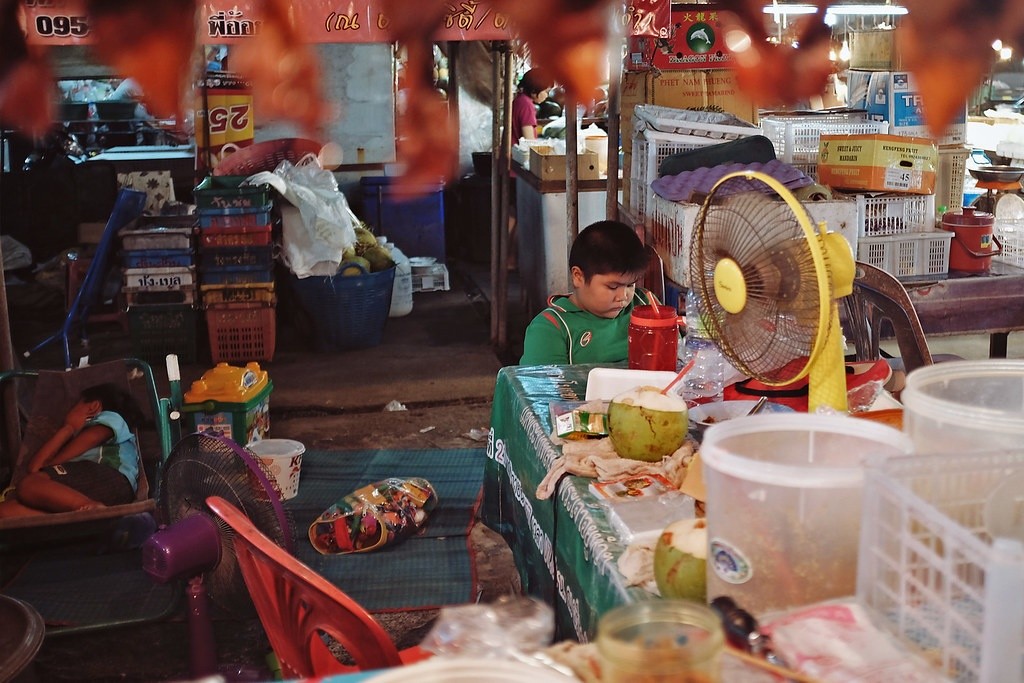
top-left (109, 175), bottom-right (282, 364)
top-left (510, 0), bottom-right (1024, 275)
top-left (176, 378), bottom-right (274, 473)
top-left (853, 442), bottom-right (1024, 683)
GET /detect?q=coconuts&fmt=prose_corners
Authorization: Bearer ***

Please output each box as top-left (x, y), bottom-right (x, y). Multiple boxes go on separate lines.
top-left (339, 228), bottom-right (391, 275)
top-left (653, 518), bottom-right (708, 604)
top-left (607, 385), bottom-right (688, 461)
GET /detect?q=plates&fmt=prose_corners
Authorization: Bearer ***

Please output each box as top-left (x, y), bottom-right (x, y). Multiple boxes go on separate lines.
top-left (408, 257), bottom-right (436, 265)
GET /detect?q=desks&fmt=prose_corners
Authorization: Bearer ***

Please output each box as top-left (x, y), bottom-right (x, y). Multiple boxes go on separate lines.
top-left (844, 259), bottom-right (1022, 357)
top-left (480, 361), bottom-right (904, 648)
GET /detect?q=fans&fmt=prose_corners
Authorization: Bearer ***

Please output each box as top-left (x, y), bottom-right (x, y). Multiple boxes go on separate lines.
top-left (138, 431), bottom-right (296, 683)
top-left (688, 171), bottom-right (858, 417)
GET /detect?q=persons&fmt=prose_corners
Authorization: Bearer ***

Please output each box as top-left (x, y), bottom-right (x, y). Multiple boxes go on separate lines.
top-left (499, 67), bottom-right (554, 274)
top-left (0, 383), bottom-right (145, 556)
top-left (519, 220), bottom-right (682, 366)
top-left (536, 86), bottom-right (565, 122)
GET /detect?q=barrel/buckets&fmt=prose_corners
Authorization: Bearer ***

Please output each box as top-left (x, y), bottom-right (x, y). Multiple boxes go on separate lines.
top-left (365, 662), bottom-right (582, 682)
top-left (942, 205), bottom-right (1002, 272)
top-left (702, 421), bottom-right (913, 622)
top-left (903, 360), bottom-right (1023, 540)
top-left (246, 441), bottom-right (305, 499)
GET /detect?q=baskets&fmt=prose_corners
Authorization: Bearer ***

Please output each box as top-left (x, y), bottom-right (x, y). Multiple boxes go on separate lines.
top-left (855, 464), bottom-right (1024, 683)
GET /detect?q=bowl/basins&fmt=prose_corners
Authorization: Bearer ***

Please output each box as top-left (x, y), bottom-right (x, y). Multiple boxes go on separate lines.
top-left (687, 398), bottom-right (795, 434)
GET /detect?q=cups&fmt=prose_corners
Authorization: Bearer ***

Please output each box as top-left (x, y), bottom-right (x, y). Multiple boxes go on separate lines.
top-left (629, 305), bottom-right (679, 372)
top-left (598, 598), bottom-right (723, 683)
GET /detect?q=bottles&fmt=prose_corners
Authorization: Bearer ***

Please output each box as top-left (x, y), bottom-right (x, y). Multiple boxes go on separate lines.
top-left (375, 236), bottom-right (413, 316)
top-left (89, 101), bottom-right (97, 121)
top-left (685, 264), bottom-right (725, 405)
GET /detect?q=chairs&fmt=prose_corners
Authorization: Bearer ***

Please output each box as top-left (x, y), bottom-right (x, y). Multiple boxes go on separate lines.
top-left (844, 260), bottom-right (966, 376)
top-left (0, 359), bottom-right (180, 637)
top-left (205, 495), bottom-right (462, 683)
